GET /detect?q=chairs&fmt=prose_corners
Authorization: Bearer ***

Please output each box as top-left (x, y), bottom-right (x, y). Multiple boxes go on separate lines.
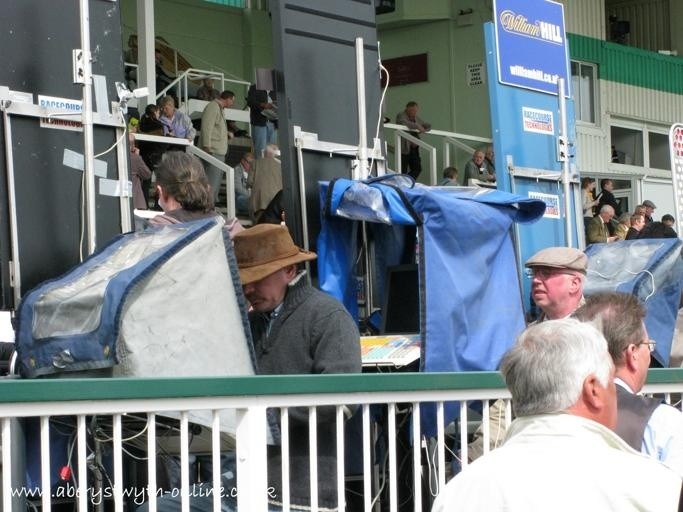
top-left (345, 403), bottom-right (383, 512)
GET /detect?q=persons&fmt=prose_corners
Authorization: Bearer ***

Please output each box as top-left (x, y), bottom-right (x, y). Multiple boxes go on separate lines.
top-left (431, 318), bottom-right (683, 512)
top-left (397, 101), bottom-right (431, 183)
top-left (438, 166), bottom-right (461, 186)
top-left (464, 150), bottom-right (493, 186)
top-left (522, 246), bottom-right (588, 325)
top-left (581, 177), bottom-right (677, 243)
top-left (483, 146), bottom-right (496, 183)
top-left (562, 291), bottom-right (683, 474)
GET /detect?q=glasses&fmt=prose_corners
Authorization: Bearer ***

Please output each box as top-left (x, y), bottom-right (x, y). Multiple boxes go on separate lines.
top-left (524, 268), bottom-right (577, 280)
top-left (632, 338), bottom-right (657, 352)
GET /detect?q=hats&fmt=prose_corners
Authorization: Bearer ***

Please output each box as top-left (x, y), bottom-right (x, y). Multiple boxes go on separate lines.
top-left (231, 223), bottom-right (317, 287)
top-left (523, 245), bottom-right (590, 277)
top-left (643, 200), bottom-right (657, 209)
